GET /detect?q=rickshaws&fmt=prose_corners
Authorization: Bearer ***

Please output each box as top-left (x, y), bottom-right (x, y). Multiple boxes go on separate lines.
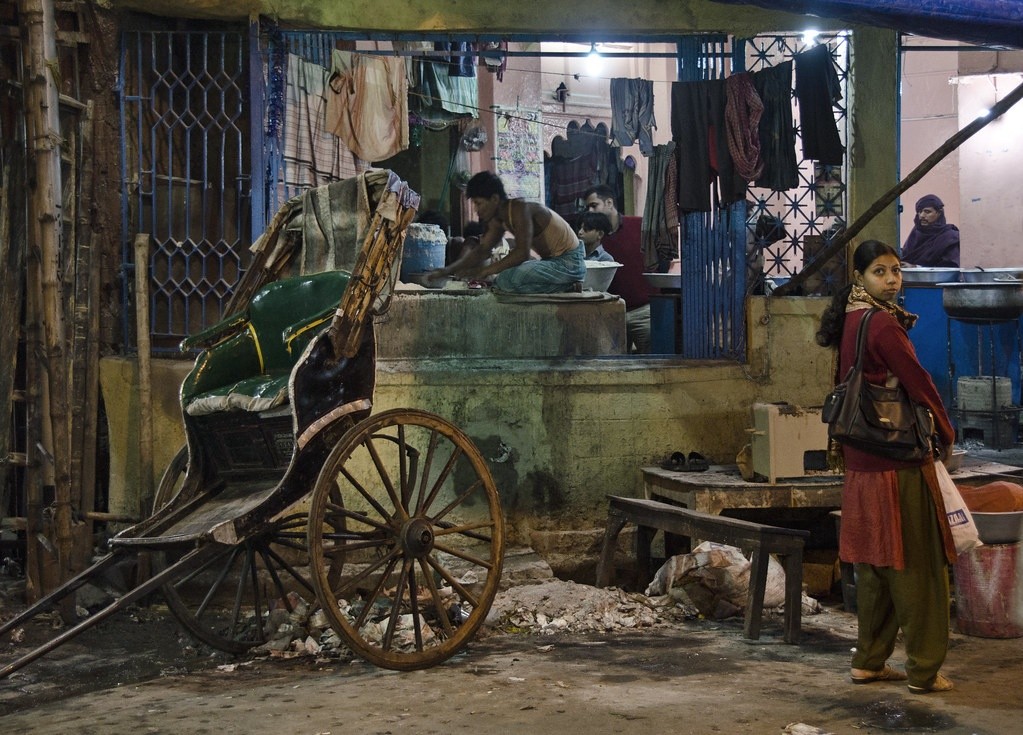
top-left (1, 167), bottom-right (506, 677)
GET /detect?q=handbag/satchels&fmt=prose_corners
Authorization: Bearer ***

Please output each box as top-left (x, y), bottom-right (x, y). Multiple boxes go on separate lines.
top-left (933, 460), bottom-right (984, 556)
top-left (821, 308), bottom-right (934, 463)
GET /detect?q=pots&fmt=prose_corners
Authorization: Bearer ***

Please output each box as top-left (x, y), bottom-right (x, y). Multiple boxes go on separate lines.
top-left (960, 268), bottom-right (1023, 281)
top-left (935, 280), bottom-right (1023, 325)
top-left (899, 266), bottom-right (963, 282)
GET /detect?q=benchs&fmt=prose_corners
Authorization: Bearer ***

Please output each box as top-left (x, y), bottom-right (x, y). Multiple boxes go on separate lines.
top-left (596, 495), bottom-right (810, 645)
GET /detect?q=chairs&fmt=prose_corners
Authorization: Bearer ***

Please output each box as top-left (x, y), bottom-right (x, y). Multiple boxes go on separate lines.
top-left (180, 269), bottom-right (353, 412)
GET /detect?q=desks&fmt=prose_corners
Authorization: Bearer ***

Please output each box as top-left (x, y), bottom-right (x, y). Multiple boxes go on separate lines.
top-left (637, 457), bottom-right (1023, 591)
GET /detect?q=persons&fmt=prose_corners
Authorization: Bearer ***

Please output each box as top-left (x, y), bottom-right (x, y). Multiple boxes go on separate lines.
top-left (428, 171), bottom-right (585, 292)
top-left (577, 213), bottom-right (614, 262)
top-left (902, 194), bottom-right (961, 266)
top-left (418, 213), bottom-right (464, 267)
top-left (814, 239), bottom-right (956, 690)
top-left (456, 222), bottom-right (509, 283)
top-left (582, 185), bottom-right (650, 354)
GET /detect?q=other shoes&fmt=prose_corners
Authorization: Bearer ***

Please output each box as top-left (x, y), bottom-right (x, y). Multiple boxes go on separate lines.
top-left (572, 281), bottom-right (584, 294)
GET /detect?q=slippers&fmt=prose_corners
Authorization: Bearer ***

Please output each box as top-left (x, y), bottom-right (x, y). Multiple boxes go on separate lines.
top-left (660, 451), bottom-right (688, 471)
top-left (850, 664), bottom-right (907, 684)
top-left (687, 451), bottom-right (709, 471)
top-left (908, 674), bottom-right (954, 692)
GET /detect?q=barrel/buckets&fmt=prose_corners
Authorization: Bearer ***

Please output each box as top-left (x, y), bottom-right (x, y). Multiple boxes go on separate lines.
top-left (646, 292), bottom-right (684, 355)
top-left (955, 374), bottom-right (1013, 411)
top-left (400, 222), bottom-right (447, 283)
top-left (954, 542), bottom-right (1023, 639)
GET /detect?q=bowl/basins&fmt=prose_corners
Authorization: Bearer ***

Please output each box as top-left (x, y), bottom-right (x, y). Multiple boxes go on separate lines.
top-left (940, 449), bottom-right (969, 473)
top-left (966, 509), bottom-right (1022, 542)
top-left (581, 259), bottom-right (624, 293)
top-left (764, 276), bottom-right (791, 288)
top-left (640, 273), bottom-right (681, 288)
top-left (408, 272), bottom-right (449, 288)
